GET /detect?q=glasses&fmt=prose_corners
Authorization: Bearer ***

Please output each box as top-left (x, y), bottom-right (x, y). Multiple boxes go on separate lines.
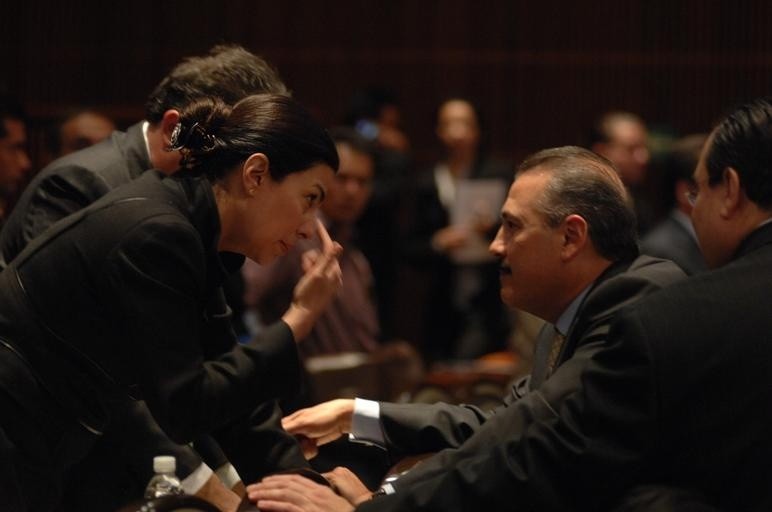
top-left (686, 183), bottom-right (702, 207)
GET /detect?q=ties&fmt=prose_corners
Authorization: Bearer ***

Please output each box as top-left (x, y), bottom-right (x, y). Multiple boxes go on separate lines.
top-left (543, 329), bottom-right (562, 381)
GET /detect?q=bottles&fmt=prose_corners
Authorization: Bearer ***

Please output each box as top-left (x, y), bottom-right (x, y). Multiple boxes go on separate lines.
top-left (140, 456), bottom-right (184, 512)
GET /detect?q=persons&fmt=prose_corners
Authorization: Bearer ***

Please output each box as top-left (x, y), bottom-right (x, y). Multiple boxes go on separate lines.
top-left (1, 103), bottom-right (119, 229)
top-left (227, 86), bottom-right (712, 494)
top-left (581, 100), bottom-right (771, 512)
top-left (1, 94), bottom-right (343, 512)
top-left (246, 146), bottom-right (689, 512)
top-left (1, 45), bottom-right (291, 512)
